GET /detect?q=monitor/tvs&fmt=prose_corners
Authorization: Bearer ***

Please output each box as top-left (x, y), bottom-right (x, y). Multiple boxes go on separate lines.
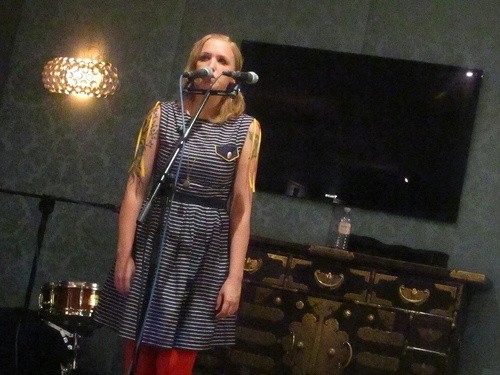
top-left (235, 40), bottom-right (484, 223)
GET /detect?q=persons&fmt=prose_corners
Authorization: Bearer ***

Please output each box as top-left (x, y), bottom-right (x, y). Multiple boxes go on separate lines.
top-left (92, 32), bottom-right (262, 375)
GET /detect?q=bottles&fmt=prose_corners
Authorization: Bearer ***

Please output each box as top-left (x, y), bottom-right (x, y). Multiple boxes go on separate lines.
top-left (331, 207), bottom-right (352, 250)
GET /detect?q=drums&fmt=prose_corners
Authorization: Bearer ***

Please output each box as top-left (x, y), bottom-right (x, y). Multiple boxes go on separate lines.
top-left (37, 279), bottom-right (108, 324)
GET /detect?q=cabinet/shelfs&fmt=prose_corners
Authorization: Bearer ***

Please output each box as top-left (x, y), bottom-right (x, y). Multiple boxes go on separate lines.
top-left (196, 233), bottom-right (494, 375)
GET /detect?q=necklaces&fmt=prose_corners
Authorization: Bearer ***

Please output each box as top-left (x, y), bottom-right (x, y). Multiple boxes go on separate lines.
top-left (183, 99), bottom-right (218, 189)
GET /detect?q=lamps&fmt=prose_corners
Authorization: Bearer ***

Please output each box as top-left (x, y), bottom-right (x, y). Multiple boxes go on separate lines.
top-left (41, 57), bottom-right (122, 98)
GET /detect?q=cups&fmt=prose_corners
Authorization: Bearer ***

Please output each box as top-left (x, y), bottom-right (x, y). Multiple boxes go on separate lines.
top-left (38, 280), bottom-right (101, 318)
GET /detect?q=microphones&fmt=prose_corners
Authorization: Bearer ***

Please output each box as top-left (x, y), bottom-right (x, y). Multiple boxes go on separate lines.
top-left (223, 71), bottom-right (259, 84)
top-left (183, 67), bottom-right (213, 79)
